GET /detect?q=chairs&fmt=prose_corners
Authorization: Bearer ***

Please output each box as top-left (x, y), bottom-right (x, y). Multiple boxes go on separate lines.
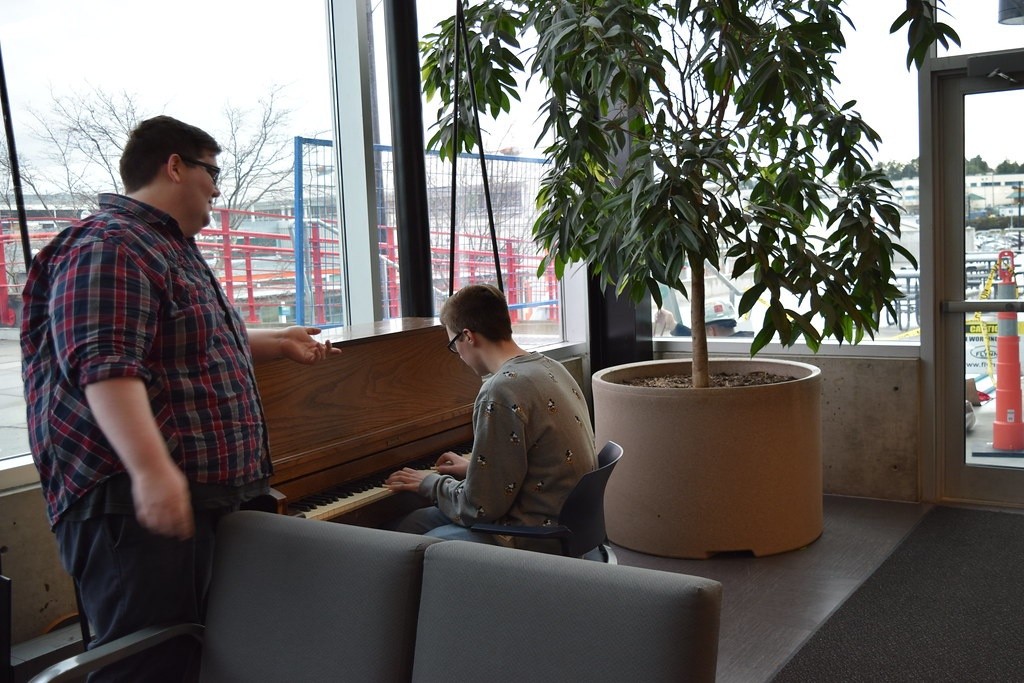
top-left (471, 440), bottom-right (625, 565)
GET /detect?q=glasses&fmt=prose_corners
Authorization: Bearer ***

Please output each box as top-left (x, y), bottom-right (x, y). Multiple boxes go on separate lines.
top-left (164, 153), bottom-right (220, 187)
top-left (446, 327), bottom-right (476, 354)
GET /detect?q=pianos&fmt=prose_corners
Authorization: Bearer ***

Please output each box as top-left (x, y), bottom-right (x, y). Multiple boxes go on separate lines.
top-left (237, 318), bottom-right (510, 531)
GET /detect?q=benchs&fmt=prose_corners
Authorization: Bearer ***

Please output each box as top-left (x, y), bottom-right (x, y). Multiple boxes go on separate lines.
top-left (964, 264), bottom-right (1024, 291)
top-left (882, 284), bottom-right (921, 331)
top-left (23, 508), bottom-right (723, 683)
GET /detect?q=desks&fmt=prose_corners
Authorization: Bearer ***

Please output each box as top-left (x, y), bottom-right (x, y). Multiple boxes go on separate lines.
top-left (883, 271), bottom-right (922, 291)
top-left (963, 258), bottom-right (999, 272)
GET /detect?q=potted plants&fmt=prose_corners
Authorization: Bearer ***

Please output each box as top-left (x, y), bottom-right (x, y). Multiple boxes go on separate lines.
top-left (414, 0), bottom-right (961, 557)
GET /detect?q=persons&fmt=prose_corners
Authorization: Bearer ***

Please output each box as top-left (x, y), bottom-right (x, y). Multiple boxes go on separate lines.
top-left (384, 284), bottom-right (601, 556)
top-left (654, 297), bottom-right (754, 337)
top-left (18, 116), bottom-right (342, 683)
